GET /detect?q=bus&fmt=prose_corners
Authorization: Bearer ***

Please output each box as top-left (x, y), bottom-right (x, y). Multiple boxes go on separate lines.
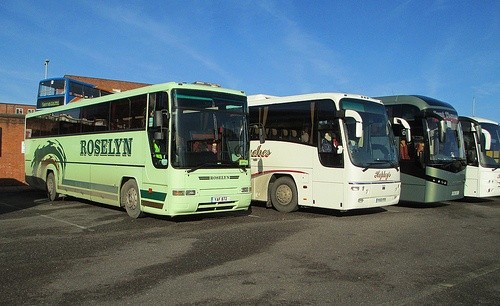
top-left (407, 114), bottom-right (500, 198)
top-left (24, 81), bottom-right (266, 220)
top-left (37, 77), bottom-right (116, 111)
top-left (124, 93), bottom-right (411, 214)
top-left (320, 94), bottom-right (483, 204)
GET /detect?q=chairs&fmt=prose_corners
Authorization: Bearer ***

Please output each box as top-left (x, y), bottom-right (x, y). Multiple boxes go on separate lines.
top-left (248, 127), bottom-right (309, 144)
top-left (31, 121), bottom-right (109, 136)
